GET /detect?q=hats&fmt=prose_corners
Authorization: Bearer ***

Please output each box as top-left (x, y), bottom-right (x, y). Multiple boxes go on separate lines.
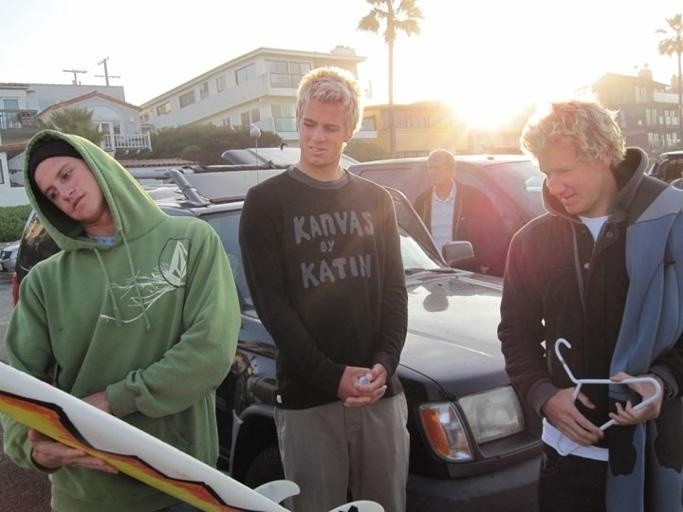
top-left (28, 138), bottom-right (82, 182)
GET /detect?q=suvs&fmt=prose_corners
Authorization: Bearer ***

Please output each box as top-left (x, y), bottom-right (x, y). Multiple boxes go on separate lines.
top-left (10, 166), bottom-right (579, 512)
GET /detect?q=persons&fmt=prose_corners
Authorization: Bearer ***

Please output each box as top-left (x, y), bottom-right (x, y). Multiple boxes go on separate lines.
top-left (413, 149), bottom-right (504, 276)
top-left (0, 129), bottom-right (242, 512)
top-left (497, 94), bottom-right (682, 512)
top-left (236, 65), bottom-right (412, 512)
